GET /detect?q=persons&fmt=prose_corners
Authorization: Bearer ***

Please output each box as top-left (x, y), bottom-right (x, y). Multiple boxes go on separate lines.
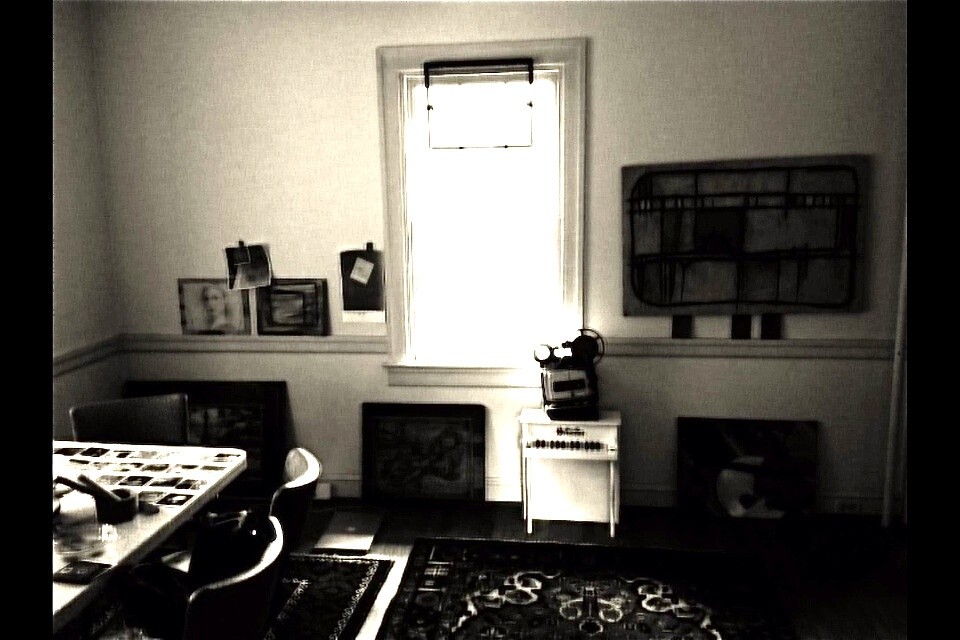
top-left (200, 286), bottom-right (237, 330)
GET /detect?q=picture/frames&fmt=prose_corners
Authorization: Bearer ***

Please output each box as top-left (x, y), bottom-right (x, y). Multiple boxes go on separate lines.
top-left (178, 279), bottom-right (251, 334)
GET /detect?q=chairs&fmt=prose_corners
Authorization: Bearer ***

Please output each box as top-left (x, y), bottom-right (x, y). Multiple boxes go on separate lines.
top-left (72, 393), bottom-right (325, 640)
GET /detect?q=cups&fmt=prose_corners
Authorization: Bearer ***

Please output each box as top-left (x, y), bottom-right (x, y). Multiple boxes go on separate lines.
top-left (52, 498), bottom-right (101, 560)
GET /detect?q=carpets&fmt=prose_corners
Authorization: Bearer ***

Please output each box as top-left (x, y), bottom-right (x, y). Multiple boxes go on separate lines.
top-left (262, 556), bottom-right (394, 639)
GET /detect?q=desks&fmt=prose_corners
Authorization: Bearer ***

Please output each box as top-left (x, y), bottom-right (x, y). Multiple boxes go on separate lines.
top-left (520, 408), bottom-right (622, 538)
top-left (52, 440), bottom-right (250, 637)
